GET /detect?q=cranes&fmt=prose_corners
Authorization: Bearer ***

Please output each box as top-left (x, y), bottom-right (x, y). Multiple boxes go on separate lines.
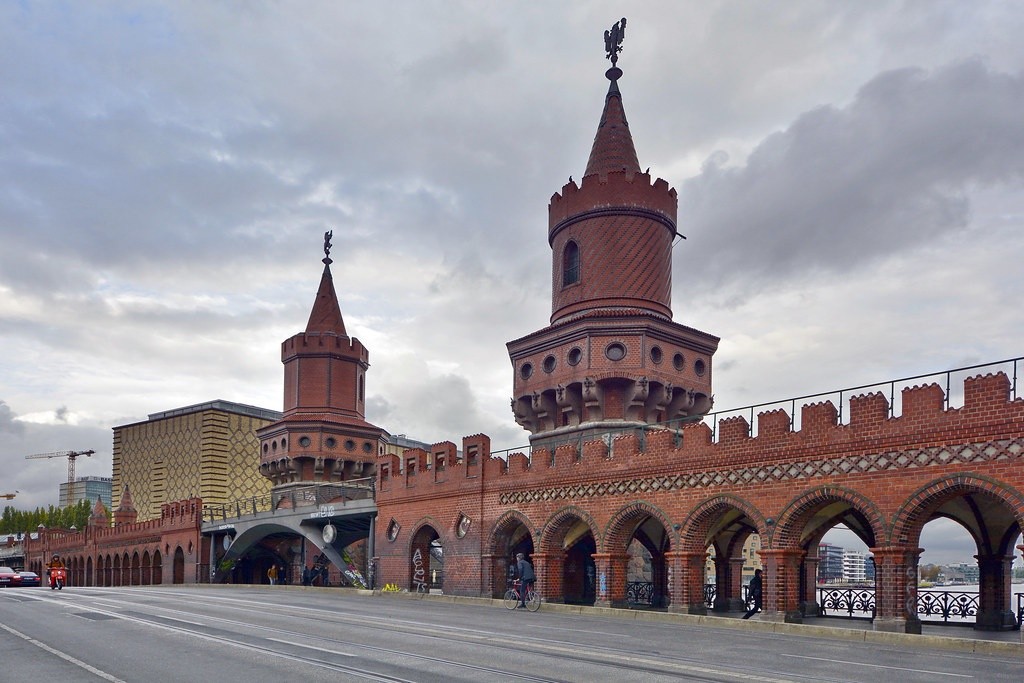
top-left (25, 449), bottom-right (96, 508)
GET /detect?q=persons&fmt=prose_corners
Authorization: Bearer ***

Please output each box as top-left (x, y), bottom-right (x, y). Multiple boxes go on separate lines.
top-left (512, 552), bottom-right (535, 608)
top-left (47, 554), bottom-right (64, 584)
top-left (267, 565), bottom-right (312, 586)
top-left (742, 569), bottom-right (763, 620)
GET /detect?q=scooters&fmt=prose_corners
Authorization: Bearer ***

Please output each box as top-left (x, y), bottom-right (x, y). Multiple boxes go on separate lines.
top-left (46, 566), bottom-right (67, 590)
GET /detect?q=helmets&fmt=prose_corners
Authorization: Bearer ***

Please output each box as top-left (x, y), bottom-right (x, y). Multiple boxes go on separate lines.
top-left (53, 554), bottom-right (60, 558)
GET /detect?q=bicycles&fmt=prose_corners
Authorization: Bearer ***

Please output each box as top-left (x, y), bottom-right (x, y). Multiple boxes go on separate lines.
top-left (504, 578), bottom-right (541, 612)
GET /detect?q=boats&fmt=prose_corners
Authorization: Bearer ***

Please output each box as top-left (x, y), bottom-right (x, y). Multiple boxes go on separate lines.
top-left (949, 577), bottom-right (967, 585)
top-left (936, 573), bottom-right (946, 586)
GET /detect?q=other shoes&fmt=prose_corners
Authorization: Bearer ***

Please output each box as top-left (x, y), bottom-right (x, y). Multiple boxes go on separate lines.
top-left (518, 604), bottom-right (526, 608)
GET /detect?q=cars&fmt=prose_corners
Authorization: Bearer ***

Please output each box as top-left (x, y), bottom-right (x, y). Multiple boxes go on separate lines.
top-left (0, 566), bottom-right (17, 588)
top-left (4, 571), bottom-right (41, 588)
